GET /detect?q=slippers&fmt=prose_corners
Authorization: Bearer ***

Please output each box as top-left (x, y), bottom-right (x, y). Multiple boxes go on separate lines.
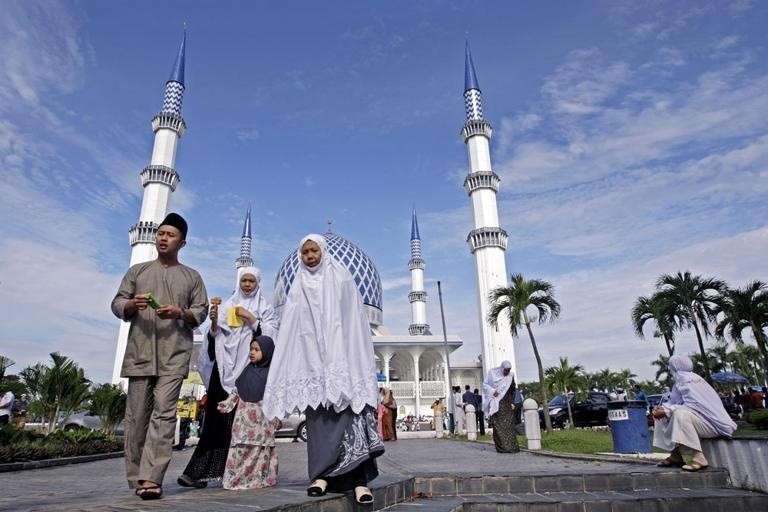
top-left (137, 484), bottom-right (162, 500)
top-left (656, 457), bottom-right (686, 467)
top-left (353, 485), bottom-right (374, 505)
top-left (307, 478), bottom-right (328, 497)
top-left (681, 459), bottom-right (709, 472)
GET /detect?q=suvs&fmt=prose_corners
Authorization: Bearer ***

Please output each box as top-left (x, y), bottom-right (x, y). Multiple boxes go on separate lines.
top-left (538, 390), bottom-right (617, 430)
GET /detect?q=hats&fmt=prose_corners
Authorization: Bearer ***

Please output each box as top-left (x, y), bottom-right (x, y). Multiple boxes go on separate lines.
top-left (159, 212), bottom-right (188, 240)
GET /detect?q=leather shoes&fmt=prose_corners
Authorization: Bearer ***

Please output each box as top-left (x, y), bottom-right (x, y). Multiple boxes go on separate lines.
top-left (176, 473), bottom-right (194, 487)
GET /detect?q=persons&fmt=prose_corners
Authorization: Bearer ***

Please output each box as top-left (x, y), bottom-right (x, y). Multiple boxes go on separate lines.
top-left (447, 385), bottom-right (485, 436)
top-left (431, 400), bottom-right (446, 438)
top-left (378, 387), bottom-right (398, 442)
top-left (11, 393), bottom-right (30, 430)
top-left (215, 333), bottom-right (283, 490)
top-left (663, 386), bottom-right (672, 402)
top-left (177, 267), bottom-right (278, 487)
top-left (616, 388), bottom-right (628, 401)
top-left (651, 353), bottom-right (737, 471)
top-left (712, 382), bottom-right (768, 420)
top-left (0, 386), bottom-right (14, 430)
top-left (262, 233), bottom-right (384, 504)
top-left (482, 361), bottom-right (524, 453)
top-left (109, 223), bottom-right (210, 500)
top-left (632, 385), bottom-right (646, 401)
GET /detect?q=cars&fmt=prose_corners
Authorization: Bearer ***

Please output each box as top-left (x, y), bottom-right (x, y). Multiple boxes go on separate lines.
top-left (646, 394), bottom-right (667, 409)
top-left (269, 411), bottom-right (308, 444)
top-left (62, 401), bottom-right (126, 438)
top-left (402, 415), bottom-right (433, 422)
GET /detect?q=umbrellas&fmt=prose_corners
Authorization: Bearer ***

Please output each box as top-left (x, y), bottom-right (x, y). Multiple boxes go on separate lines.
top-left (704, 369), bottom-right (749, 387)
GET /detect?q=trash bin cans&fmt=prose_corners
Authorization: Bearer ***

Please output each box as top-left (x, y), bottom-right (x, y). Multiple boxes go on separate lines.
top-left (607, 399), bottom-right (653, 455)
